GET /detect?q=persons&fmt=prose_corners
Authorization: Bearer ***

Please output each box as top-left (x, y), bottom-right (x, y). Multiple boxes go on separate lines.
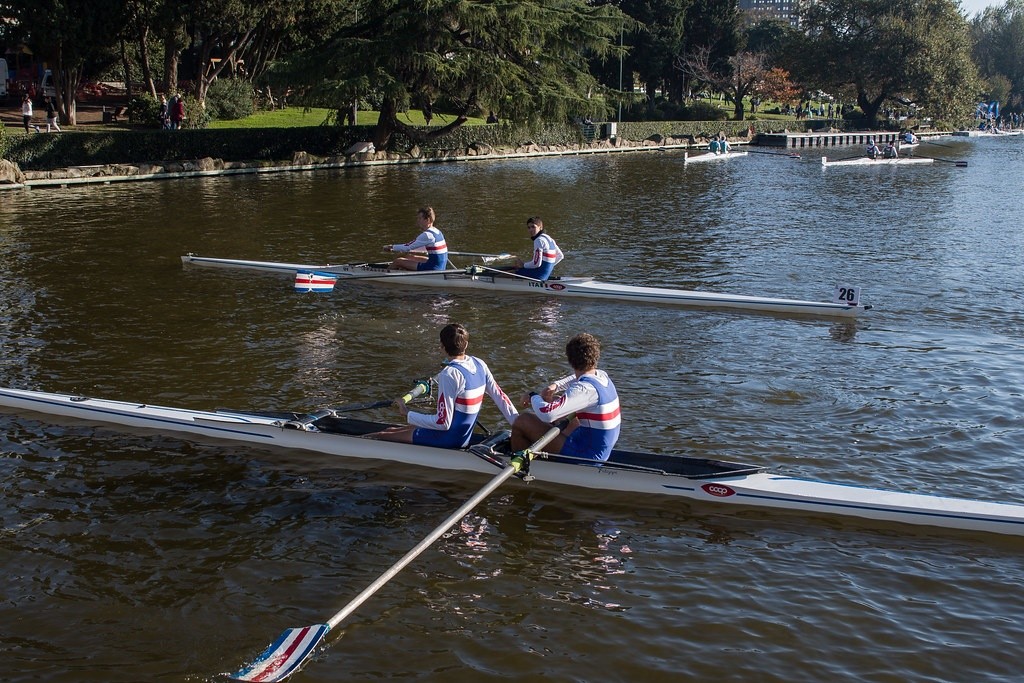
top-left (784, 102), bottom-right (855, 121)
top-left (19, 93), bottom-right (40, 133)
top-left (486, 111), bottom-right (496, 123)
top-left (510, 332), bottom-right (621, 466)
top-left (384, 206), bottom-right (448, 271)
top-left (362, 323), bottom-right (519, 449)
top-left (719, 136), bottom-right (731, 154)
top-left (44, 96), bottom-right (61, 133)
top-left (866, 140), bottom-right (881, 157)
top-left (882, 141), bottom-right (897, 159)
top-left (706, 137), bottom-right (721, 154)
top-left (495, 216), bottom-right (564, 281)
top-left (899, 129), bottom-right (916, 144)
top-left (987, 112), bottom-right (1024, 130)
top-left (159, 93), bottom-right (185, 129)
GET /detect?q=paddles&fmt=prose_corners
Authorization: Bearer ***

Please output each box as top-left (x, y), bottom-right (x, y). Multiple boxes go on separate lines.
top-left (294, 264), bottom-right (517, 293)
top-left (217, 415), bottom-right (574, 683)
top-left (395, 373), bottom-right (439, 408)
top-left (385, 244), bottom-right (518, 260)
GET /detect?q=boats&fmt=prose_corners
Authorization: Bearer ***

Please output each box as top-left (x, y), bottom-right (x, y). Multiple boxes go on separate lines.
top-left (684, 152), bottom-right (749, 162)
top-left (978, 132), bottom-right (1020, 136)
top-left (0, 387), bottom-right (1024, 537)
top-left (898, 141), bottom-right (919, 150)
top-left (182, 253), bottom-right (874, 316)
top-left (822, 156), bottom-right (935, 166)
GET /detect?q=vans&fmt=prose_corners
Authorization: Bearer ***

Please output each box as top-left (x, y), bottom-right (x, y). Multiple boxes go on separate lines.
top-left (40, 69), bottom-right (65, 98)
top-left (0, 58), bottom-right (10, 95)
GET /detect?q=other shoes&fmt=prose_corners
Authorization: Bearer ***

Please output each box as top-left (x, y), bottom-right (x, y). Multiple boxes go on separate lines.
top-left (35, 126), bottom-right (39, 132)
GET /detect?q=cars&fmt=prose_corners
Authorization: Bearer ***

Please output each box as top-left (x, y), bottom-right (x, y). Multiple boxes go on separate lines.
top-left (633, 87), bottom-right (646, 93)
